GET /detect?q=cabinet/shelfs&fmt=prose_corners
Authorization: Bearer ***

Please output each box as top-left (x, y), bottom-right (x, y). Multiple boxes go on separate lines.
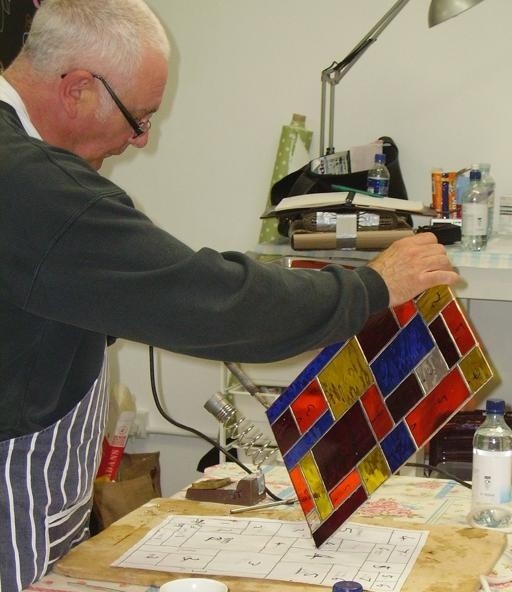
top-left (202, 252), bottom-right (427, 484)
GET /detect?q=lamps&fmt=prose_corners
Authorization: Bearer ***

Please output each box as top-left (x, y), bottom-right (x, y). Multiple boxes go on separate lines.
top-left (317, 0), bottom-right (484, 159)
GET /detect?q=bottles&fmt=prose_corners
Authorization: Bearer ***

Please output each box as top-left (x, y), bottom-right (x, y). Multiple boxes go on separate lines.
top-left (460, 161), bottom-right (497, 254)
top-left (366, 153), bottom-right (391, 197)
top-left (470, 398), bottom-right (512, 529)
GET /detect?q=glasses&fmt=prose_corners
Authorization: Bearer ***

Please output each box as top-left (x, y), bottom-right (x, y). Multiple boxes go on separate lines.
top-left (61, 72), bottom-right (152, 139)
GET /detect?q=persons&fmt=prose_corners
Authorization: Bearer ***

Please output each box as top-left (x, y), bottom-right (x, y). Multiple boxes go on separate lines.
top-left (0, 0), bottom-right (462, 592)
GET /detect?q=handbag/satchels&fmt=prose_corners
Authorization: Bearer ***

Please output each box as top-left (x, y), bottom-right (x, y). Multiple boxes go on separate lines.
top-left (270, 136), bottom-right (413, 238)
